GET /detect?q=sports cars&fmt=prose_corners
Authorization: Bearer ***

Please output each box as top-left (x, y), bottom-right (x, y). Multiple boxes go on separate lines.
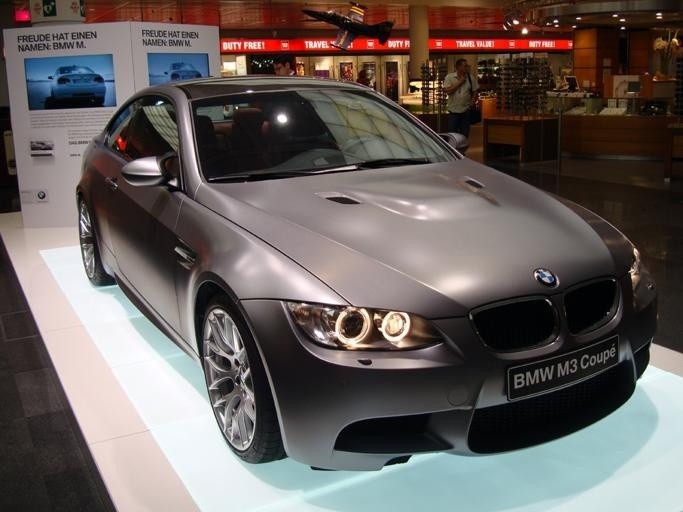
top-left (75, 75), bottom-right (658, 473)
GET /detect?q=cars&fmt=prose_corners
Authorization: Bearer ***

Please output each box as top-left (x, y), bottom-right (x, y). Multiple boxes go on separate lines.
top-left (47, 66), bottom-right (106, 105)
top-left (163, 63), bottom-right (203, 81)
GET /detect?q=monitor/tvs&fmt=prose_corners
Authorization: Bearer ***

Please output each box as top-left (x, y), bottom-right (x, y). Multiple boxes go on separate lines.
top-left (564, 76), bottom-right (579, 90)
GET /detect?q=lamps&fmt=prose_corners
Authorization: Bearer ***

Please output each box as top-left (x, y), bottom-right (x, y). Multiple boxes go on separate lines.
top-left (503, 10), bottom-right (522, 31)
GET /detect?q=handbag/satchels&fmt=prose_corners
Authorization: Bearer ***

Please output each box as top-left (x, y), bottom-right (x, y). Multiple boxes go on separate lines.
top-left (469, 105), bottom-right (481, 125)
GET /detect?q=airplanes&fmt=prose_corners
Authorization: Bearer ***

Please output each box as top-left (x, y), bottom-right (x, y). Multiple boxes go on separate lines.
top-left (301, 1), bottom-right (394, 51)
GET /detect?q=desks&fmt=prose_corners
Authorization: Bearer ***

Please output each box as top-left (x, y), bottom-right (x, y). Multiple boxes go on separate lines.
top-left (481, 113), bottom-right (558, 162)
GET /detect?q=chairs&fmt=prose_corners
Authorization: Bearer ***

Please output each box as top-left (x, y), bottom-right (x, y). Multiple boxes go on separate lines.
top-left (125, 106), bottom-right (288, 176)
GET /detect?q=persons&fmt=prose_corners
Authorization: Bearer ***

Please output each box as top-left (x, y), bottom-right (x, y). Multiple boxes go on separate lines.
top-left (273, 52), bottom-right (296, 75)
top-left (442, 58), bottom-right (482, 138)
top-left (355, 69), bottom-right (370, 87)
